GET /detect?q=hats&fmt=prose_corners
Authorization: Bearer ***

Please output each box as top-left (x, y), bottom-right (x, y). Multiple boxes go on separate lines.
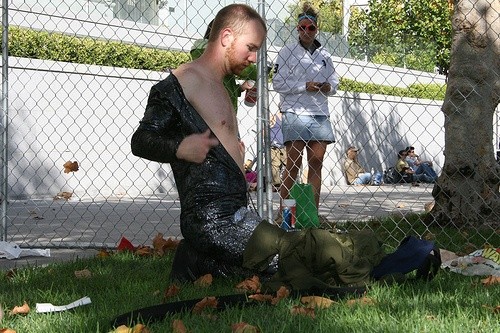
top-left (347, 147), bottom-right (360, 154)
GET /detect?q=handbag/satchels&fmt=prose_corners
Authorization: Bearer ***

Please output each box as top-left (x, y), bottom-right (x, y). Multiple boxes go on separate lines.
top-left (289, 183), bottom-right (321, 228)
top-left (385, 168), bottom-right (398, 183)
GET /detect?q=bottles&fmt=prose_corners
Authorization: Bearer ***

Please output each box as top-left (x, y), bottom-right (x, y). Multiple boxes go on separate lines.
top-left (282, 198), bottom-right (296, 230)
top-left (371, 167), bottom-right (375, 176)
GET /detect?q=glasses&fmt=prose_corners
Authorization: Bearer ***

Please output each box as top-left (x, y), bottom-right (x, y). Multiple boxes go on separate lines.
top-left (401, 153), bottom-right (407, 156)
top-left (298, 24), bottom-right (317, 32)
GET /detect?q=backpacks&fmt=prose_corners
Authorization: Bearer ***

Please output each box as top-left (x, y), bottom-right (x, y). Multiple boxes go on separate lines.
top-left (369, 235), bottom-right (442, 283)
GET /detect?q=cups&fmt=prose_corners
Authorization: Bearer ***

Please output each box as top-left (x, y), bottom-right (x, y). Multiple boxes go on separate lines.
top-left (244, 80), bottom-right (258, 107)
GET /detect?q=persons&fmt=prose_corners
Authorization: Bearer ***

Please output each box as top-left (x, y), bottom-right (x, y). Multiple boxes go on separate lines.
top-left (131, 4), bottom-right (312, 282)
top-left (404, 147), bottom-right (438, 184)
top-left (397, 150), bottom-right (421, 186)
top-left (191, 18), bottom-right (272, 142)
top-left (344, 146), bottom-right (384, 186)
top-left (273, 2), bottom-right (339, 222)
top-left (244, 155), bottom-right (257, 191)
top-left (270, 114), bottom-right (287, 192)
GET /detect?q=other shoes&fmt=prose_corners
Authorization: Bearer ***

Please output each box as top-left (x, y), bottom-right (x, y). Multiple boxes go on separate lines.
top-left (169, 239), bottom-right (195, 283)
top-left (371, 181), bottom-right (384, 186)
top-left (411, 182), bottom-right (419, 186)
